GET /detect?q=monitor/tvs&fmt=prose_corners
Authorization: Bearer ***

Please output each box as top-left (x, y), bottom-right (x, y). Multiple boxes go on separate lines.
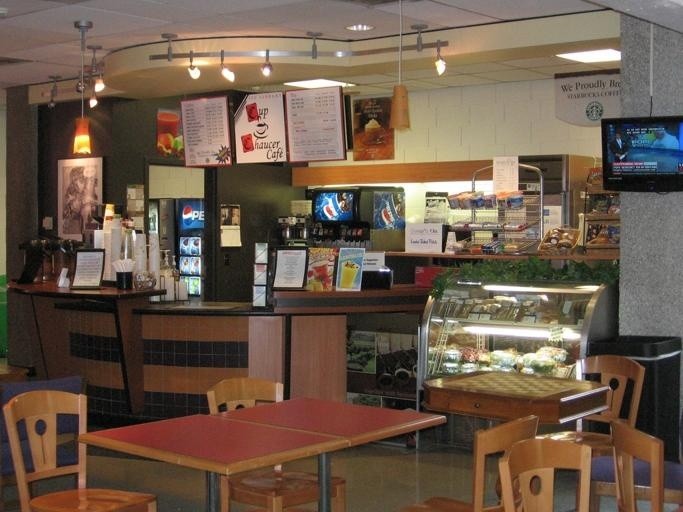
top-left (600, 116), bottom-right (683, 192)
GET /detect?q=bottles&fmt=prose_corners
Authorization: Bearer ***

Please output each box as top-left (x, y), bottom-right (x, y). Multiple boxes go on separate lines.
top-left (159, 250), bottom-right (174, 277)
top-left (170, 255), bottom-right (180, 283)
top-left (119, 228), bottom-right (135, 260)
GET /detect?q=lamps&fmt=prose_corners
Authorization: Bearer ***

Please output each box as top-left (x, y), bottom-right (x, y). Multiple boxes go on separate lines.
top-left (556, 47), bottom-right (621, 66)
top-left (73, 19), bottom-right (93, 156)
top-left (260, 48), bottom-right (274, 79)
top-left (186, 49), bottom-right (201, 80)
top-left (281, 78), bottom-right (358, 91)
top-left (89, 79), bottom-right (99, 109)
top-left (94, 62), bottom-right (106, 93)
top-left (435, 39), bottom-right (447, 78)
top-left (220, 49), bottom-right (236, 82)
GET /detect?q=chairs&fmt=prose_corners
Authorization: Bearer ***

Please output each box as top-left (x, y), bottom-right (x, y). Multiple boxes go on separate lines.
top-left (3, 388), bottom-right (158, 512)
top-left (497, 436), bottom-right (592, 512)
top-left (609, 418), bottom-right (682, 512)
top-left (0, 374), bottom-right (90, 511)
top-left (206, 375), bottom-right (348, 512)
top-left (398, 413), bottom-right (540, 512)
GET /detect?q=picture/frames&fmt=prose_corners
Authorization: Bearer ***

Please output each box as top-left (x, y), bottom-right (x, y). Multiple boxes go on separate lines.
top-left (53, 154), bottom-right (106, 241)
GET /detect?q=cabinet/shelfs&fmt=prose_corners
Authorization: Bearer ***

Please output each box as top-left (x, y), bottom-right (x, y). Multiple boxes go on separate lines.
top-left (447, 164), bottom-right (544, 254)
top-left (583, 169), bottom-right (621, 254)
top-left (419, 273), bottom-right (615, 424)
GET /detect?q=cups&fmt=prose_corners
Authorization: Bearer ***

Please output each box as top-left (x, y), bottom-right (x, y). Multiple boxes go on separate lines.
top-left (339, 260), bottom-right (360, 288)
top-left (120, 229), bottom-right (133, 259)
top-left (116, 272), bottom-right (132, 290)
top-left (111, 213), bottom-right (122, 281)
top-left (103, 204), bottom-right (114, 280)
top-left (148, 232), bottom-right (161, 302)
top-left (135, 234), bottom-right (147, 274)
top-left (93, 229), bottom-right (104, 248)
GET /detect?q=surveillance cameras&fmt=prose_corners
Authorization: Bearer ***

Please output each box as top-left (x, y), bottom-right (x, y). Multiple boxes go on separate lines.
top-left (47, 101), bottom-right (56, 110)
top-left (76, 83), bottom-right (86, 92)
